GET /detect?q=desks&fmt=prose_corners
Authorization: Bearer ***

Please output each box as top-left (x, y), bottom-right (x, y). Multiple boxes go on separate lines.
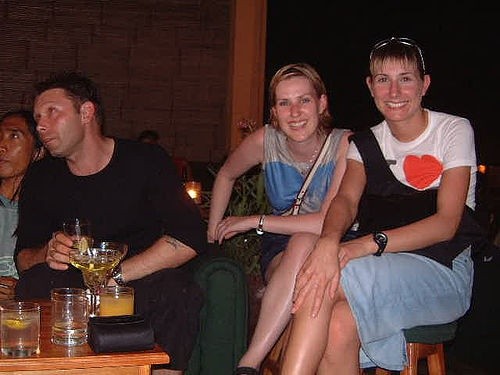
top-left (0, 300), bottom-right (170, 375)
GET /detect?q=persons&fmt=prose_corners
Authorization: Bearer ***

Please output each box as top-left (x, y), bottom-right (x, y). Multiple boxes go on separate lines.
top-left (139, 130), bottom-right (160, 144)
top-left (0, 110), bottom-right (48, 300)
top-left (280, 38), bottom-right (478, 375)
top-left (205, 63), bottom-right (354, 375)
top-left (14, 71), bottom-right (207, 375)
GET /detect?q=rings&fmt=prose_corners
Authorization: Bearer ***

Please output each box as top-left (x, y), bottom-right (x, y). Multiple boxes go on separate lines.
top-left (55, 232), bottom-right (62, 239)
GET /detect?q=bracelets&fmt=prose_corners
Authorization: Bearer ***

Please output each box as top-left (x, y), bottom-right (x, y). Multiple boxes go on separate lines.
top-left (257, 214), bottom-right (266, 235)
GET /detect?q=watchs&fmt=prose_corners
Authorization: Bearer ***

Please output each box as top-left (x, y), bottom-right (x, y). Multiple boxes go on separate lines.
top-left (373, 231), bottom-right (388, 256)
top-left (111, 264), bottom-right (125, 286)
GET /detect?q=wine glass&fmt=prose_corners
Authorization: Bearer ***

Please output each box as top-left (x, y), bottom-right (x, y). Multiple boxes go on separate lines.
top-left (68, 239), bottom-right (127, 318)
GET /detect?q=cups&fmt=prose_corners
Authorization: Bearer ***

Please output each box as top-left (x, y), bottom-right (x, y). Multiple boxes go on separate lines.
top-left (63, 220), bottom-right (93, 254)
top-left (100, 286), bottom-right (134, 317)
top-left (51, 289), bottom-right (90, 347)
top-left (0, 303), bottom-right (41, 358)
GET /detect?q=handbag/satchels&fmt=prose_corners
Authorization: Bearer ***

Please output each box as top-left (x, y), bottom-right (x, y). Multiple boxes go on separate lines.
top-left (347, 128), bottom-right (488, 269)
top-left (84, 314), bottom-right (155, 353)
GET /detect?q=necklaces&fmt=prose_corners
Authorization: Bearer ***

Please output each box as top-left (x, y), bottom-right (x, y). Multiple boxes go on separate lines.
top-left (301, 139), bottom-right (321, 174)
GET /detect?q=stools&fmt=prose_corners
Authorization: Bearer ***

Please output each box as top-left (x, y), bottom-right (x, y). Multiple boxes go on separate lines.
top-left (376, 322), bottom-right (458, 375)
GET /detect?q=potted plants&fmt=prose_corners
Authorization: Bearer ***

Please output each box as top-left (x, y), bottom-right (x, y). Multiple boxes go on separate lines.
top-left (204, 155), bottom-right (272, 349)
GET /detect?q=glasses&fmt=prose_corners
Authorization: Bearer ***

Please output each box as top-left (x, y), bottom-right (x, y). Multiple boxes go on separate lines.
top-left (370, 36), bottom-right (426, 75)
